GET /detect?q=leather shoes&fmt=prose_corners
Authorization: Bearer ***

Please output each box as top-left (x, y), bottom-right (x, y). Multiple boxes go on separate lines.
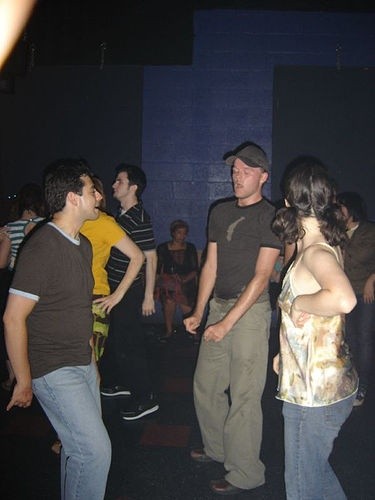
top-left (191, 449), bottom-right (212, 462)
top-left (210, 478), bottom-right (245, 495)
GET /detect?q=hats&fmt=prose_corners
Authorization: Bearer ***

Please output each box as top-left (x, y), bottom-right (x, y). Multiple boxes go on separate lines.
top-left (226, 144), bottom-right (270, 172)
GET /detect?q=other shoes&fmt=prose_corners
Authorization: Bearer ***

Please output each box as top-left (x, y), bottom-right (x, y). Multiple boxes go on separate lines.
top-left (158, 328), bottom-right (177, 343)
top-left (100, 385), bottom-right (131, 396)
top-left (353, 384), bottom-right (368, 406)
top-left (2, 381), bottom-right (12, 391)
top-left (121, 400), bottom-right (159, 421)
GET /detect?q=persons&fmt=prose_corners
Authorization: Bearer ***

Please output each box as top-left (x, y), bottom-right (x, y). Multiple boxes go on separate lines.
top-left (182, 144), bottom-right (281, 496)
top-left (0, 183), bottom-right (46, 391)
top-left (154, 220), bottom-right (199, 343)
top-left (3, 161), bottom-right (111, 500)
top-left (100, 164), bottom-right (160, 420)
top-left (272, 156), bottom-right (359, 500)
top-left (52, 178), bottom-right (146, 452)
top-left (338, 191), bottom-right (375, 405)
